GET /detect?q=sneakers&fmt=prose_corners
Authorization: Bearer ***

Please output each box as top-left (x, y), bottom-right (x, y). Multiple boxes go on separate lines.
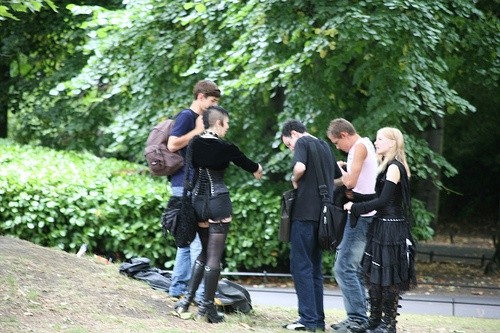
top-left (283, 321), bottom-right (309, 331)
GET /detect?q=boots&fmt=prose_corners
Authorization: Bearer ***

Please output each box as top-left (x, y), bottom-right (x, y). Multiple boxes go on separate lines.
top-left (174, 260), bottom-right (206, 313)
top-left (346, 289), bottom-right (383, 333)
top-left (366, 292), bottom-right (403, 333)
top-left (198, 266), bottom-right (224, 323)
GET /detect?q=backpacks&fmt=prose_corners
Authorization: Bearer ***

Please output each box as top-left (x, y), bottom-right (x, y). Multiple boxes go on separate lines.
top-left (144, 118), bottom-right (184, 176)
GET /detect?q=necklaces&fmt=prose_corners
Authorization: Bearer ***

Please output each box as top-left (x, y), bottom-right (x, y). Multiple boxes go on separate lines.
top-left (201, 131), bottom-right (218, 138)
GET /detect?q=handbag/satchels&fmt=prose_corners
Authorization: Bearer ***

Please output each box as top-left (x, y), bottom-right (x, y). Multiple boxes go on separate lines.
top-left (163, 197), bottom-right (197, 248)
top-left (318, 204), bottom-right (347, 250)
top-left (279, 189), bottom-right (298, 241)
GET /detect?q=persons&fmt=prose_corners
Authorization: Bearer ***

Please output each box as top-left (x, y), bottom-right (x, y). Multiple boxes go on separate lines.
top-left (327, 117), bottom-right (378, 333)
top-left (280, 121), bottom-right (342, 333)
top-left (343, 127), bottom-right (418, 333)
top-left (167, 79), bottom-right (234, 307)
top-left (173, 106), bottom-right (262, 322)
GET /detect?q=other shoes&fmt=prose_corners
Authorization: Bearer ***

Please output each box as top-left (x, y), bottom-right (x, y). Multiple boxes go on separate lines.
top-left (330, 317), bottom-right (353, 331)
top-left (172, 295), bottom-right (198, 306)
